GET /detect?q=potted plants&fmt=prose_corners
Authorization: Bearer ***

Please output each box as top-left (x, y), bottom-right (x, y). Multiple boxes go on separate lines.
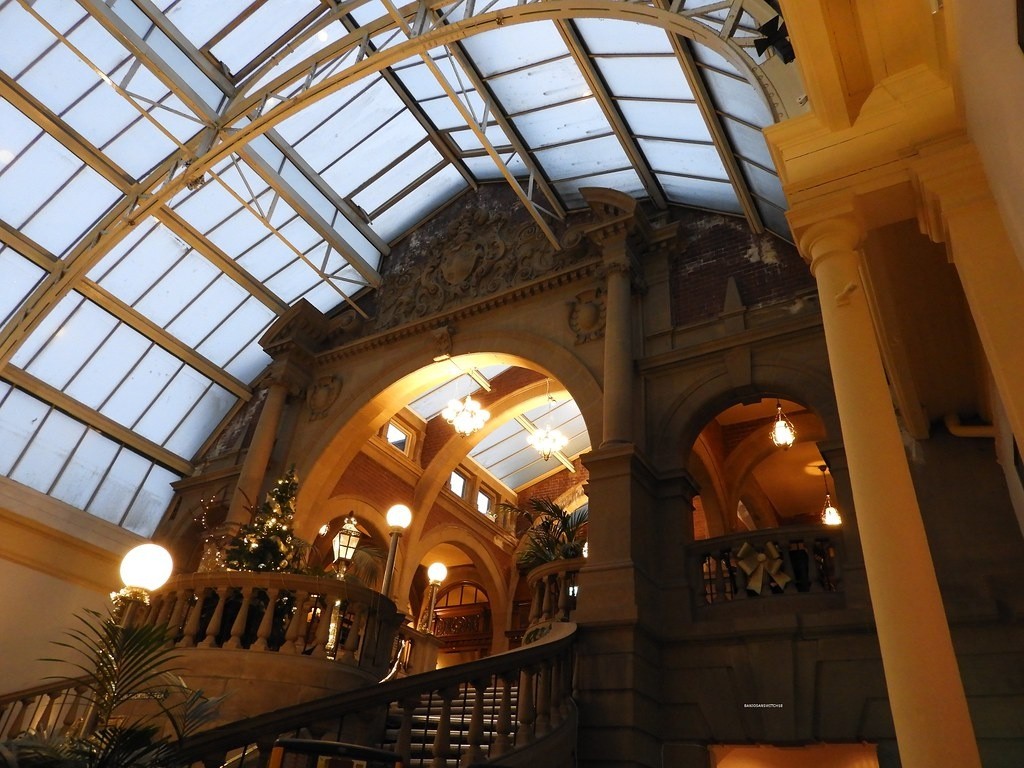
top-left (494, 495), bottom-right (588, 622)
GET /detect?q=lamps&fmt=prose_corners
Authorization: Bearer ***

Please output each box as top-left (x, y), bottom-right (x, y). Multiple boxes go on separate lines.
top-left (526, 399), bottom-right (569, 461)
top-left (427, 563), bottom-right (447, 588)
top-left (120, 544), bottom-right (173, 600)
top-left (819, 465), bottom-right (842, 525)
top-left (386, 504), bottom-right (412, 536)
top-left (442, 367), bottom-right (490, 438)
top-left (768, 397), bottom-right (798, 450)
top-left (332, 511), bottom-right (360, 582)
